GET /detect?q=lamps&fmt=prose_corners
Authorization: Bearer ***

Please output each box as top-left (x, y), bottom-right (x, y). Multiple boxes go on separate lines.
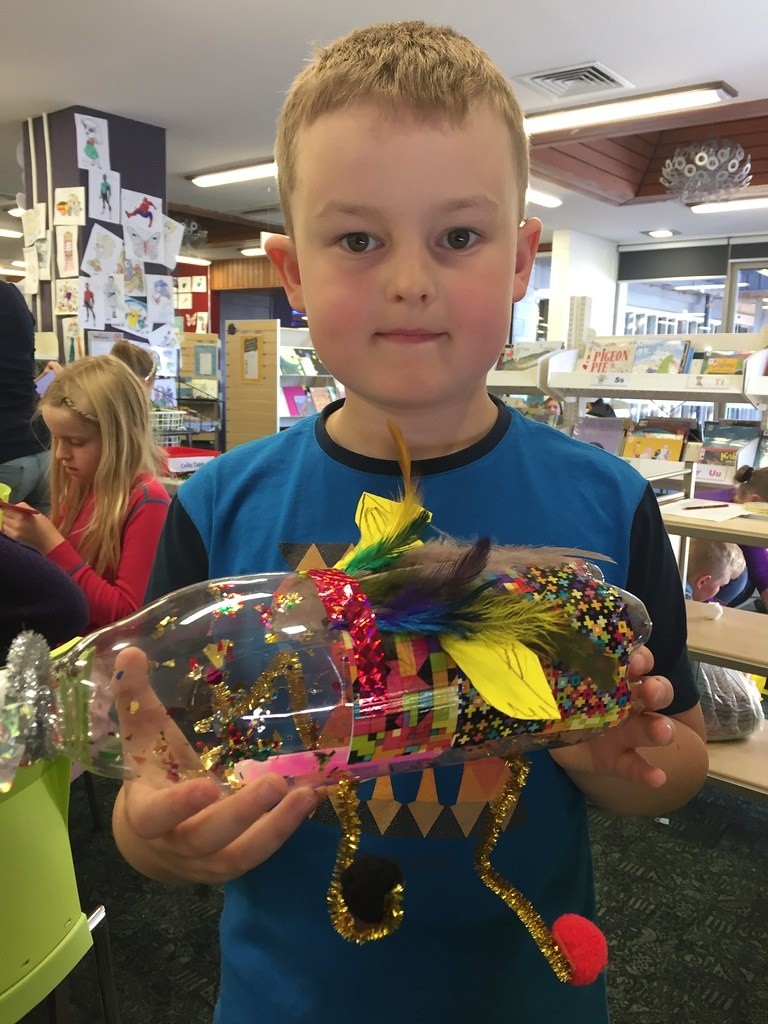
top-left (237, 245), bottom-right (267, 256)
top-left (685, 194), bottom-right (768, 214)
top-left (183, 81), bottom-right (739, 189)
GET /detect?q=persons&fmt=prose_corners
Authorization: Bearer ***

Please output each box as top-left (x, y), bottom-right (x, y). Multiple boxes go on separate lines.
top-left (542, 397), bottom-right (564, 427)
top-left (0, 227), bottom-right (226, 1023)
top-left (683, 465), bottom-right (768, 698)
top-left (113, 23), bottom-right (708, 1023)
top-left (587, 396), bottom-right (617, 418)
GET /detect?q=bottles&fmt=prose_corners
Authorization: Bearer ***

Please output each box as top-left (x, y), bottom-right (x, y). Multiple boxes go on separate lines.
top-left (0, 562), bottom-right (653, 791)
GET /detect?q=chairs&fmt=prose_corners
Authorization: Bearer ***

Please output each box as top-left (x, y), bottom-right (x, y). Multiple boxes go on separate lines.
top-left (1, 753), bottom-right (120, 1024)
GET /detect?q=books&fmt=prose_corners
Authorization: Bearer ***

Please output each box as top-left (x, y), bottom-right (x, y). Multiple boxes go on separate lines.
top-left (276, 334), bottom-right (768, 504)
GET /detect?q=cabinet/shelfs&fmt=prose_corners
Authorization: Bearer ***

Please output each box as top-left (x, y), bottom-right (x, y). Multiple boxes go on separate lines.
top-left (175, 332), bottom-right (226, 451)
top-left (226, 319), bottom-right (768, 806)
